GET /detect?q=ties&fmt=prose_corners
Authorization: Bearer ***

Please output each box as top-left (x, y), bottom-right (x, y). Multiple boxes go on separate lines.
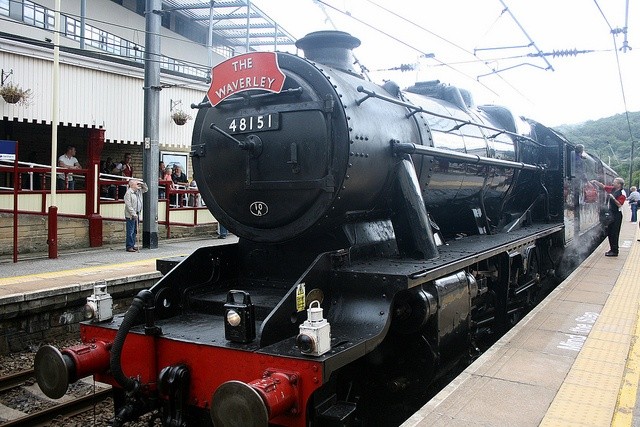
top-left (104, 166), bottom-right (109, 174)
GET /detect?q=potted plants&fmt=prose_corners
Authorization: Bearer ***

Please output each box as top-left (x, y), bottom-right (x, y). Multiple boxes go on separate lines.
top-left (0, 84), bottom-right (32, 107)
top-left (170, 106), bottom-right (192, 125)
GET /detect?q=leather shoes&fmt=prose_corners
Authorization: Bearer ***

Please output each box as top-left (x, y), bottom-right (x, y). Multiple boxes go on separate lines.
top-left (128, 248), bottom-right (136, 252)
top-left (133, 248), bottom-right (138, 250)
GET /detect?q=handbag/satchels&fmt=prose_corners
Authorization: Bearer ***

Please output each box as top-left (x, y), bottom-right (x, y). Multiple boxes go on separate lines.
top-left (101, 187), bottom-right (108, 194)
top-left (599, 208), bottom-right (615, 226)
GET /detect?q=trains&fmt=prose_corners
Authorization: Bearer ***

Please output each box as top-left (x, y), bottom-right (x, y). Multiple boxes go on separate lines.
top-left (34, 30), bottom-right (620, 426)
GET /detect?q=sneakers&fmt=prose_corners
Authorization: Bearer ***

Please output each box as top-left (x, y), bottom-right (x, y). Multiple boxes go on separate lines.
top-left (218, 235), bottom-right (225, 239)
top-left (605, 252), bottom-right (617, 256)
top-left (606, 250), bottom-right (615, 253)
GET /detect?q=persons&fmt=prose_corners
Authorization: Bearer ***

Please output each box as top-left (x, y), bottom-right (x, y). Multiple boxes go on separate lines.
top-left (159, 160), bottom-right (165, 198)
top-left (590, 177), bottom-right (627, 256)
top-left (625, 185), bottom-right (640, 222)
top-left (171, 165), bottom-right (188, 208)
top-left (190, 173), bottom-right (202, 206)
top-left (57, 143), bottom-right (82, 190)
top-left (123, 179), bottom-right (149, 251)
top-left (164, 167), bottom-right (177, 190)
top-left (100, 156), bottom-right (114, 172)
top-left (112, 152), bottom-right (134, 199)
